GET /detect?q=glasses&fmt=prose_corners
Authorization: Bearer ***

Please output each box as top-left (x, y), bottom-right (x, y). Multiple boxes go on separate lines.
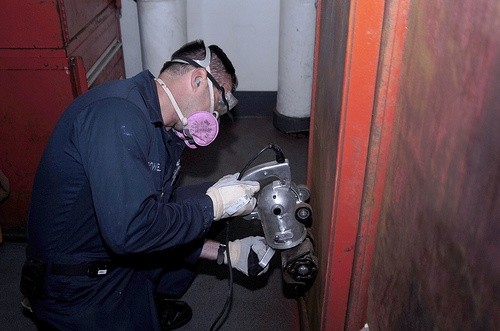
top-left (214, 89), bottom-right (238, 116)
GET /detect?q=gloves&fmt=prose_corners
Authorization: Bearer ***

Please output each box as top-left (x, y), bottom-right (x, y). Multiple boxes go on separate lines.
top-left (206, 172), bottom-right (261, 221)
top-left (223, 236), bottom-right (270, 278)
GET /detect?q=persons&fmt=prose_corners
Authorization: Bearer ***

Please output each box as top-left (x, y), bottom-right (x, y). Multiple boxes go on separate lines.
top-left (19, 39), bottom-right (269, 331)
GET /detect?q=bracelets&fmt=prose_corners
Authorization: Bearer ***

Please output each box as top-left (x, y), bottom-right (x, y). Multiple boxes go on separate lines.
top-left (216, 243), bottom-right (227, 265)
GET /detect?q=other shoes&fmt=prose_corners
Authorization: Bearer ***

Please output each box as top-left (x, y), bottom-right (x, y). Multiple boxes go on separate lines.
top-left (160, 299), bottom-right (192, 329)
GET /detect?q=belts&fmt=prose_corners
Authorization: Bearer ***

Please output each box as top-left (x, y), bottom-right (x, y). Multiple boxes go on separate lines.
top-left (54, 260), bottom-right (117, 278)
